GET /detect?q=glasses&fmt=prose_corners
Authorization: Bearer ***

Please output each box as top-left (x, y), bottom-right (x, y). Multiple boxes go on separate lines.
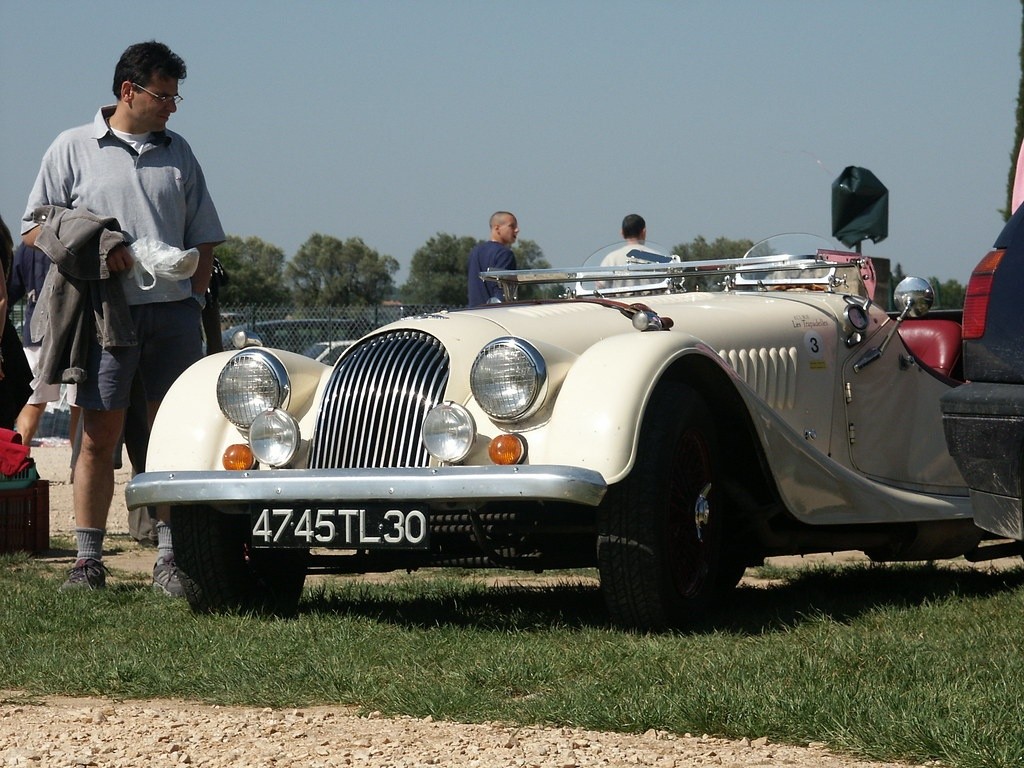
top-left (131, 82), bottom-right (184, 106)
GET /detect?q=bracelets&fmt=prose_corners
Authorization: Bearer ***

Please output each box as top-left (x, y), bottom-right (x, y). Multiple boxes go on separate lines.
top-left (192, 293), bottom-right (206, 310)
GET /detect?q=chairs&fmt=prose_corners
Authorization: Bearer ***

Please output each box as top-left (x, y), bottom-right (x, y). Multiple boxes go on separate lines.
top-left (896, 320), bottom-right (964, 378)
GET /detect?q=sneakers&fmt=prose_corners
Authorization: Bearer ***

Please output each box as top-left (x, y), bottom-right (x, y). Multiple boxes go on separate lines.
top-left (151, 553), bottom-right (185, 597)
top-left (56, 557), bottom-right (113, 592)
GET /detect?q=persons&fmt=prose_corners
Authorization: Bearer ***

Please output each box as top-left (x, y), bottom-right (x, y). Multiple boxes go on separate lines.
top-left (468, 211), bottom-right (520, 307)
top-left (0, 219), bottom-right (160, 551)
top-left (19, 42), bottom-right (228, 596)
top-left (595, 212), bottom-right (670, 297)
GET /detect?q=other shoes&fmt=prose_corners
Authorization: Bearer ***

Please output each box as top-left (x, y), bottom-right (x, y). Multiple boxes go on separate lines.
top-left (139, 537), bottom-right (158, 550)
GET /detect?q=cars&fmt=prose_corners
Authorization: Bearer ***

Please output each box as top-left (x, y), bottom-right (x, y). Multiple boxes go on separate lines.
top-left (937, 193), bottom-right (1024, 545)
top-left (121, 227), bottom-right (977, 635)
